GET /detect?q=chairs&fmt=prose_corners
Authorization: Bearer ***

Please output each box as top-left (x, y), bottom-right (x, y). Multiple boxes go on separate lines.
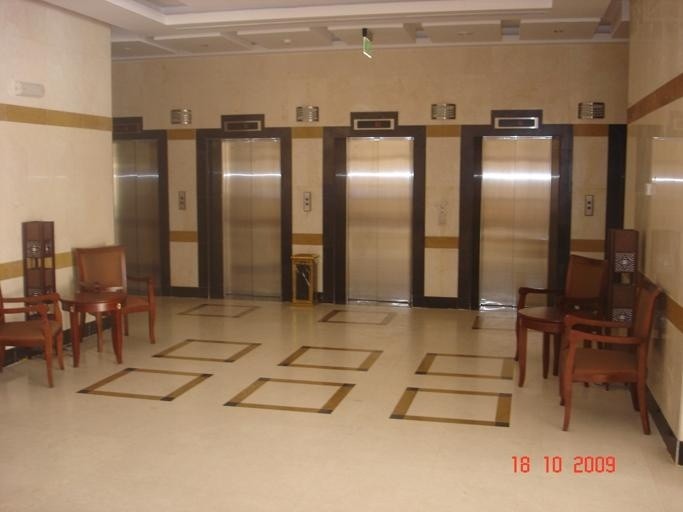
top-left (513, 254), bottom-right (663, 434)
top-left (0, 243), bottom-right (156, 388)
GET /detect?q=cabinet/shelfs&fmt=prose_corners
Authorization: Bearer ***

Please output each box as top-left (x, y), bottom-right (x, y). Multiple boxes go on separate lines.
top-left (20, 219), bottom-right (57, 359)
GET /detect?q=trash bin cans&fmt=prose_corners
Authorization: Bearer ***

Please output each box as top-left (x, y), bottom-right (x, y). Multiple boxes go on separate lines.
top-left (291, 253), bottom-right (319, 305)
